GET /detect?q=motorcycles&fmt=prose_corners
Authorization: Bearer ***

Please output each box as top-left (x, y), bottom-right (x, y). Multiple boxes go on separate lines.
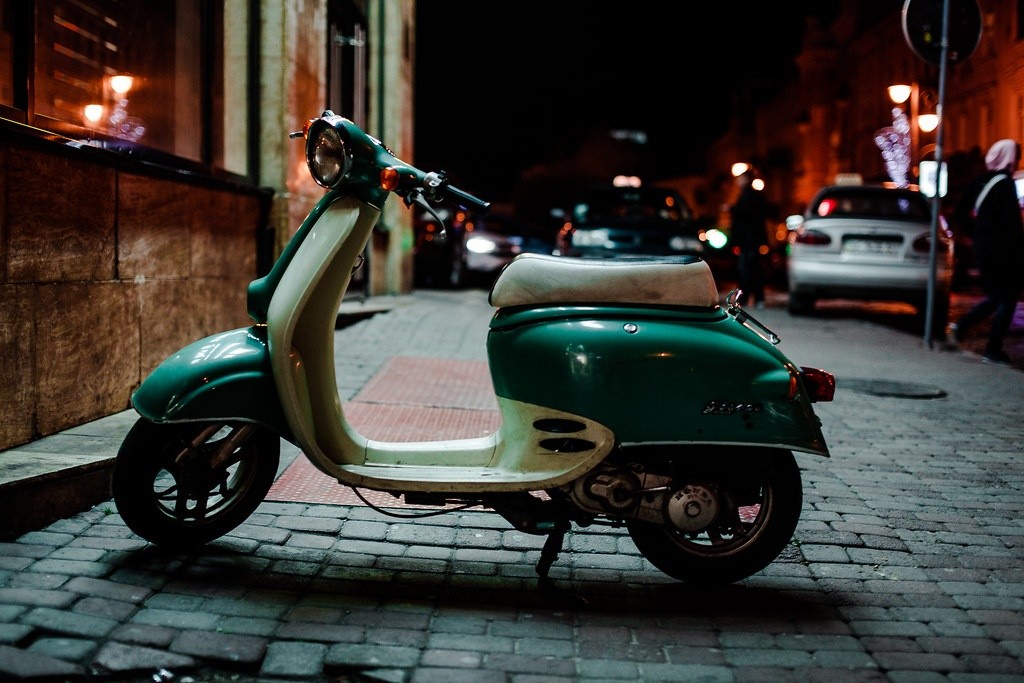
top-left (108, 108), bottom-right (837, 580)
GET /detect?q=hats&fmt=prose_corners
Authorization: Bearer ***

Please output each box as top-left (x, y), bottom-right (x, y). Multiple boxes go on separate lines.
top-left (985, 138), bottom-right (1015, 173)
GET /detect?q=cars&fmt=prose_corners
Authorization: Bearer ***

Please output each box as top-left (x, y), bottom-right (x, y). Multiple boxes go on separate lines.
top-left (784, 182), bottom-right (954, 322)
top-left (449, 214), bottom-right (523, 288)
top-left (544, 182), bottom-right (711, 272)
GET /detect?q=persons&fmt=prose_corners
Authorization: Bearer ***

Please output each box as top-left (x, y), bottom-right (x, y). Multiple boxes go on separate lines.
top-left (945, 139), bottom-right (1023, 364)
top-left (729, 171), bottom-right (782, 310)
